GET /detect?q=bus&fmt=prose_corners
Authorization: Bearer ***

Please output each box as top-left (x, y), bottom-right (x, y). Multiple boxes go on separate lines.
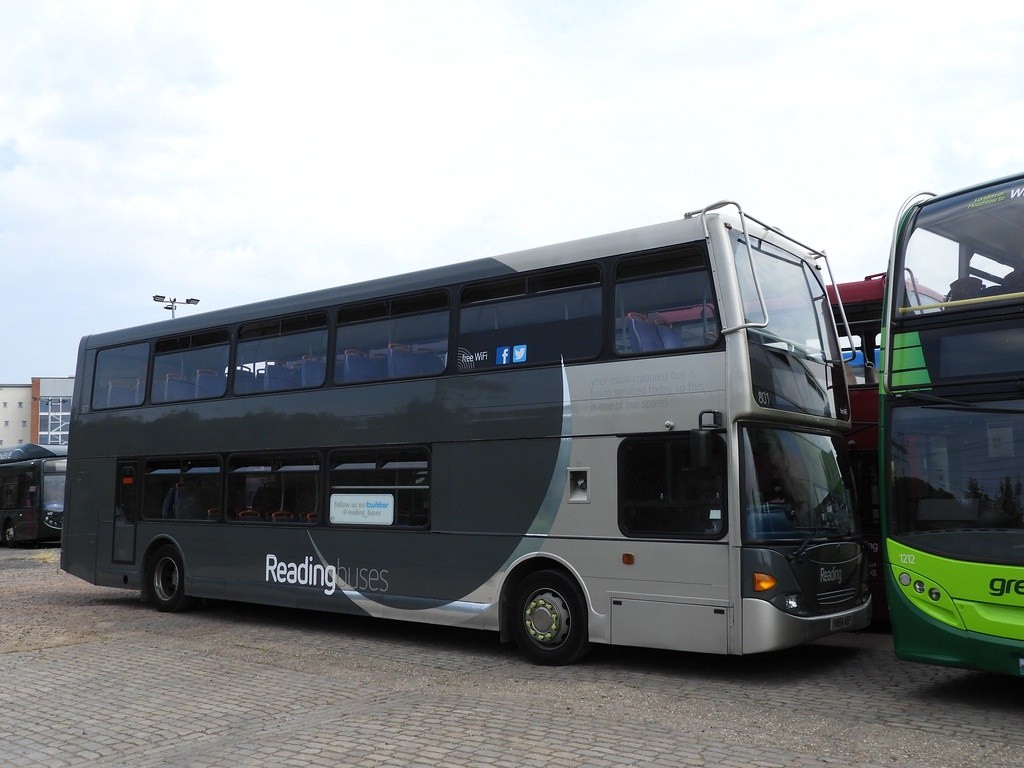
top-left (878, 171), bottom-right (1024, 684)
top-left (0, 442), bottom-right (72, 549)
top-left (57, 198), bottom-right (877, 668)
top-left (607, 267), bottom-right (958, 632)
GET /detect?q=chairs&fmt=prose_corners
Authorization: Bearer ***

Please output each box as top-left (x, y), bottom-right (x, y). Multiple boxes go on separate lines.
top-left (106, 344), bottom-right (443, 407)
top-left (206, 508), bottom-right (322, 523)
top-left (944, 269), bottom-right (1024, 304)
